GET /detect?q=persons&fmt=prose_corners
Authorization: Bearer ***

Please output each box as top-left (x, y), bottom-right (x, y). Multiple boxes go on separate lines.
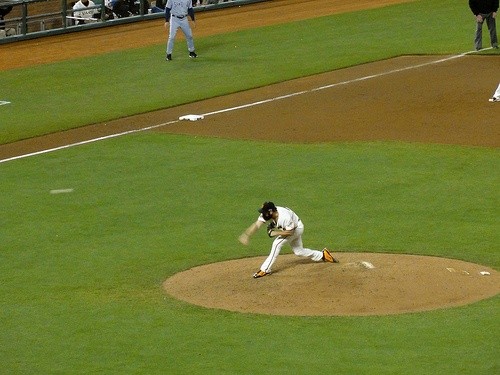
top-left (488, 82), bottom-right (500, 104)
top-left (103, 0), bottom-right (226, 19)
top-left (72, 0), bottom-right (100, 26)
top-left (468, 0), bottom-right (500, 51)
top-left (163, 0), bottom-right (199, 61)
top-left (238, 201), bottom-right (340, 279)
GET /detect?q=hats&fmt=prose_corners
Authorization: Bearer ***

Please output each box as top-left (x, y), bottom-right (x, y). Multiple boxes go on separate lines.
top-left (258, 201), bottom-right (275, 213)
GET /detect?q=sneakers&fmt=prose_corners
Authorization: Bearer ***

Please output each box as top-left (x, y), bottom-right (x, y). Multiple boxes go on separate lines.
top-left (189, 52), bottom-right (198, 57)
top-left (166, 54), bottom-right (172, 60)
top-left (488, 97), bottom-right (500, 102)
top-left (253, 270), bottom-right (272, 278)
top-left (321, 247), bottom-right (336, 262)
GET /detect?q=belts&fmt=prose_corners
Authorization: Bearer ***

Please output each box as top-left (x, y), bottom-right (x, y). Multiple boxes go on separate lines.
top-left (172, 14), bottom-right (187, 18)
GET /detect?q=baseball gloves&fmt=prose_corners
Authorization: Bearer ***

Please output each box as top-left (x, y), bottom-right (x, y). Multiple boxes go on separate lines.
top-left (266, 222), bottom-right (276, 238)
